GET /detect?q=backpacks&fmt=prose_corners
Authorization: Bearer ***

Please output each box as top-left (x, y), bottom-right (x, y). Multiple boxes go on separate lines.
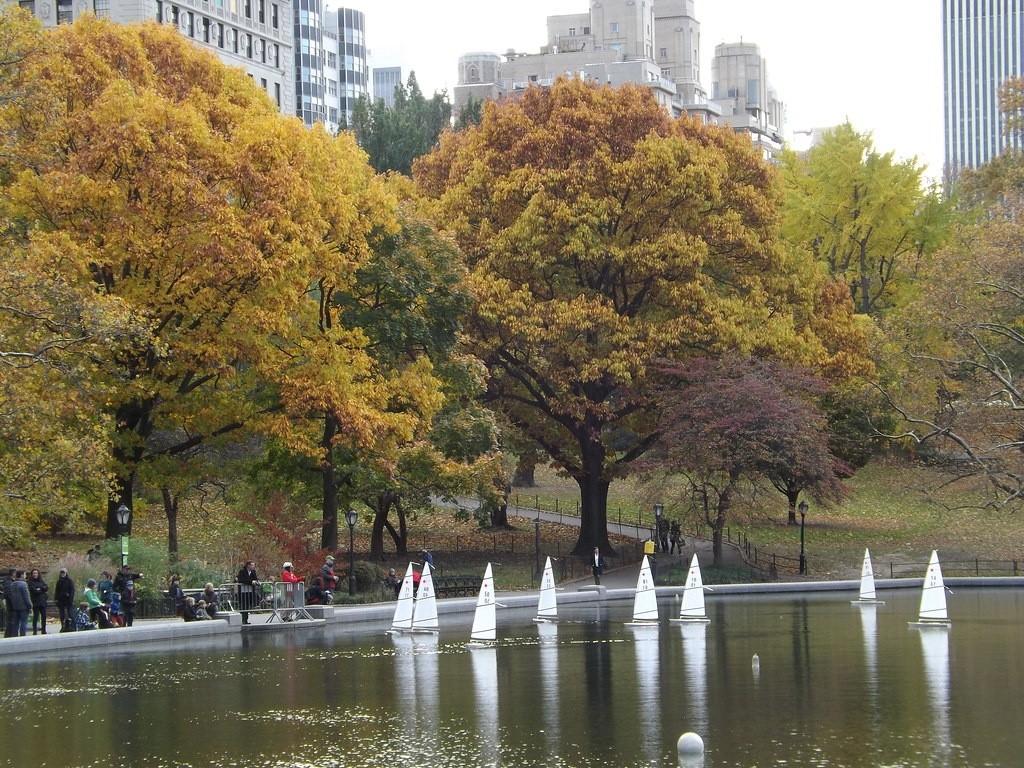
top-left (61, 618), bottom-right (76, 632)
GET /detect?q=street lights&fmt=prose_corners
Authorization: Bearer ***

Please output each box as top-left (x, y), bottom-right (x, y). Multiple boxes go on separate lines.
top-left (650, 501), bottom-right (665, 578)
top-left (798, 500), bottom-right (808, 573)
top-left (115, 502), bottom-right (132, 568)
top-left (344, 508), bottom-right (359, 598)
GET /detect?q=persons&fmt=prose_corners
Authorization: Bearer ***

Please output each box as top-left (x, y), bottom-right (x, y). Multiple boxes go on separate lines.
top-left (309, 576), bottom-right (328, 605)
top-left (183, 596), bottom-right (201, 622)
top-left (669, 520), bottom-right (684, 556)
top-left (2, 568), bottom-right (49, 638)
top-left (659, 514), bottom-right (670, 554)
top-left (112, 564), bottom-right (144, 592)
top-left (52, 567), bottom-right (77, 631)
top-left (199, 581), bottom-right (218, 617)
top-left (194, 600), bottom-right (213, 621)
top-left (84, 579), bottom-right (115, 629)
top-left (110, 593), bottom-right (126, 627)
top-left (388, 567), bottom-right (402, 590)
top-left (167, 577), bottom-right (188, 607)
top-left (590, 546), bottom-right (603, 585)
top-left (321, 555), bottom-right (339, 597)
top-left (121, 580), bottom-right (137, 627)
top-left (422, 549), bottom-right (433, 575)
top-left (237, 560), bottom-right (262, 625)
top-left (96, 570), bottom-right (112, 611)
top-left (279, 561), bottom-right (306, 622)
top-left (75, 602), bottom-right (98, 630)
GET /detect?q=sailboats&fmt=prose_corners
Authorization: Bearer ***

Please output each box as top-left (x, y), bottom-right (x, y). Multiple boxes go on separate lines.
top-left (533, 556), bottom-right (560, 625)
top-left (623, 555), bottom-right (662, 627)
top-left (667, 552), bottom-right (716, 624)
top-left (385, 560), bottom-right (442, 635)
top-left (462, 562), bottom-right (499, 647)
top-left (848, 547), bottom-right (888, 605)
top-left (907, 547), bottom-right (954, 627)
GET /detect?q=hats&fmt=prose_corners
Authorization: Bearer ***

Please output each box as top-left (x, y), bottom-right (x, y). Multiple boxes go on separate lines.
top-left (88, 579), bottom-right (97, 586)
top-left (123, 565), bottom-right (131, 570)
top-left (126, 580), bottom-right (134, 586)
top-left (100, 571), bottom-right (109, 576)
top-left (284, 562), bottom-right (294, 568)
top-left (60, 568), bottom-right (67, 573)
top-left (326, 555), bottom-right (335, 560)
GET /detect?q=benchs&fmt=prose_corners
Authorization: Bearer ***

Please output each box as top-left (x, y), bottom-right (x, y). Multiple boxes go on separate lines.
top-left (162, 591), bottom-right (238, 614)
top-left (384, 576), bottom-right (484, 600)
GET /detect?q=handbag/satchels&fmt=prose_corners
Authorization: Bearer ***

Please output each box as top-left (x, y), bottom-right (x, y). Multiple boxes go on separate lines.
top-left (675, 535), bottom-right (685, 546)
